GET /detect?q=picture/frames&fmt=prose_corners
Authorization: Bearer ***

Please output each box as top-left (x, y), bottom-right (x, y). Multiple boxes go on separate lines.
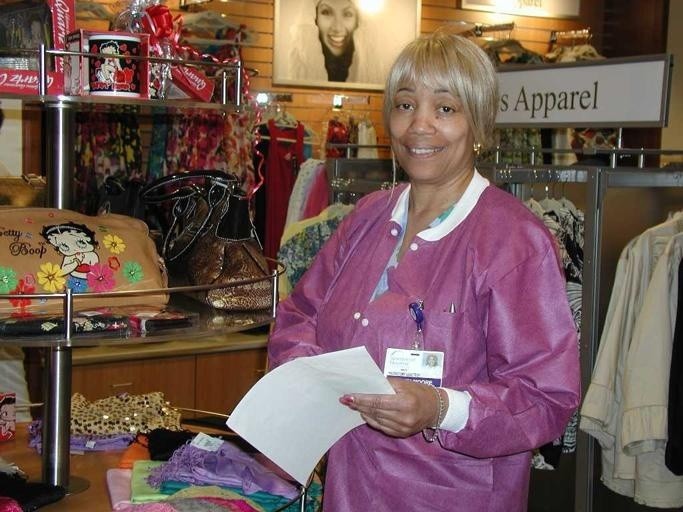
top-left (270, 0), bottom-right (423, 93)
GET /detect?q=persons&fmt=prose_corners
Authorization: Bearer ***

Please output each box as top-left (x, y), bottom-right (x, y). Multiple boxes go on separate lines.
top-left (267, 31), bottom-right (581, 512)
top-left (315, 0), bottom-right (359, 81)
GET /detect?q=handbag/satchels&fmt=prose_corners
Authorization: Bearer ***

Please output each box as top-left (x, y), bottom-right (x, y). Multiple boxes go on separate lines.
top-left (139, 171), bottom-right (279, 310)
top-left (0, 207), bottom-right (170, 318)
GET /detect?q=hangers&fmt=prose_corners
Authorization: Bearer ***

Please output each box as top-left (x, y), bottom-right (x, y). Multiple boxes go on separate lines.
top-left (243, 103), bottom-right (321, 147)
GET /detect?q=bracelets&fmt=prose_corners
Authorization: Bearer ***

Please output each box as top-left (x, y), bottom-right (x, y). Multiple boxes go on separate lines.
top-left (422, 384), bottom-right (446, 442)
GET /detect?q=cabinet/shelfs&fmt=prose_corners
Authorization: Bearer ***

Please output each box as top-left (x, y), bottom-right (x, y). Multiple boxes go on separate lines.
top-left (1, 41), bottom-right (323, 511)
top-left (197, 348), bottom-right (268, 422)
top-left (69, 351), bottom-right (195, 424)
